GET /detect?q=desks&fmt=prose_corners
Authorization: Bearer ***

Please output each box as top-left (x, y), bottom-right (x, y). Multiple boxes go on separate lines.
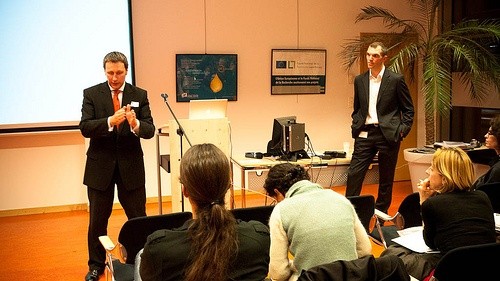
top-left (229, 154), bottom-right (379, 210)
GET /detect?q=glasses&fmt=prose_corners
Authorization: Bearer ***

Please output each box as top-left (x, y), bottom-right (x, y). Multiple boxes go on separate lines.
top-left (487, 129), bottom-right (494, 135)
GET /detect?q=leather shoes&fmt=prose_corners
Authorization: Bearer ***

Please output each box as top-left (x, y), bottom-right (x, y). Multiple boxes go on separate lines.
top-left (85, 268), bottom-right (106, 281)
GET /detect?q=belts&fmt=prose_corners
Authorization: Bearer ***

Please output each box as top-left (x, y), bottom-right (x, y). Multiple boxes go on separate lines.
top-left (363, 123), bottom-right (380, 130)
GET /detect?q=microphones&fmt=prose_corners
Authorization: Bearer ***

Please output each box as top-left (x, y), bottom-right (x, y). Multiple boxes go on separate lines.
top-left (245, 152), bottom-right (263, 159)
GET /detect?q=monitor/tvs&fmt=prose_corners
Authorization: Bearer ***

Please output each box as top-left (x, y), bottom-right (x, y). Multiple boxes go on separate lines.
top-left (272, 115), bottom-right (297, 155)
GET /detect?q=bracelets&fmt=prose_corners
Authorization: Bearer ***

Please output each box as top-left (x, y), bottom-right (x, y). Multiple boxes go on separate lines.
top-left (419, 205), bottom-right (424, 219)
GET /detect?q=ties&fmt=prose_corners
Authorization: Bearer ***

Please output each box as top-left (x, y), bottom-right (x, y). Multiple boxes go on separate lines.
top-left (113, 91), bottom-right (122, 131)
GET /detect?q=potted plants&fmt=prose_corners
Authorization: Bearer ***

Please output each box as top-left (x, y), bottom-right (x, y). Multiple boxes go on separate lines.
top-left (337, 0), bottom-right (500, 206)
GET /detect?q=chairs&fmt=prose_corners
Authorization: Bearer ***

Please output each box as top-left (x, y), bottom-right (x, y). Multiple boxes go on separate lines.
top-left (156, 126), bottom-right (170, 215)
top-left (99, 194), bottom-right (500, 281)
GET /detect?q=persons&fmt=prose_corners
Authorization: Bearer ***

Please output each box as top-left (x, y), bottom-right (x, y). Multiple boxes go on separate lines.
top-left (419, 146), bottom-right (495, 280)
top-left (469, 117), bottom-right (500, 213)
top-left (263, 162), bottom-right (372, 281)
top-left (139, 143), bottom-right (270, 281)
top-left (79, 52), bottom-right (155, 281)
top-left (345, 41), bottom-right (414, 229)
